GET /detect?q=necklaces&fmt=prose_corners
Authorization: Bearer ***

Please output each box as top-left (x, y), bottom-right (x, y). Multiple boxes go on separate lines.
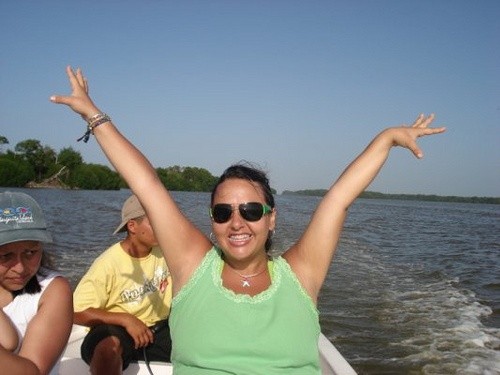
top-left (225, 262), bottom-right (268, 287)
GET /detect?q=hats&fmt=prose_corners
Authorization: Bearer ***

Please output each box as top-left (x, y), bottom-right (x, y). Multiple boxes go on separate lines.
top-left (0, 191), bottom-right (53, 246)
top-left (112, 195), bottom-right (147, 236)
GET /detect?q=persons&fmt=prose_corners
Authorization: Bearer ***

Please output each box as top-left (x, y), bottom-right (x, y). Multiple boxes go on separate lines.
top-left (74, 193), bottom-right (172, 375)
top-left (0, 191), bottom-right (74, 375)
top-left (49, 63), bottom-right (446, 375)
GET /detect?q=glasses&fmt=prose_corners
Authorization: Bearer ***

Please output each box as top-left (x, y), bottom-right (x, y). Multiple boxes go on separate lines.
top-left (208, 202), bottom-right (272, 224)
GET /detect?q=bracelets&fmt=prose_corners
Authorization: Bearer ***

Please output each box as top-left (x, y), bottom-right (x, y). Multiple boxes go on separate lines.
top-left (76, 112), bottom-right (112, 143)
